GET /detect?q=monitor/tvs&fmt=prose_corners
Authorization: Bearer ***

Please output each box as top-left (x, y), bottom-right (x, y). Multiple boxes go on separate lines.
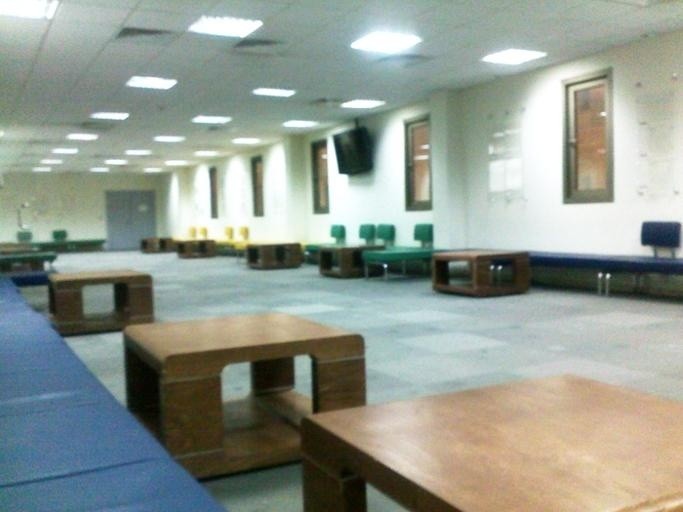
top-left (333, 126), bottom-right (372, 174)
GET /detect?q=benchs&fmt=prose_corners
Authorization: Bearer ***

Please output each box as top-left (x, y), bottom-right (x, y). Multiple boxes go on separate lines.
top-left (305, 225), bottom-right (432, 281)
top-left (492, 220), bottom-right (680, 298)
top-left (0, 269), bottom-right (226, 511)
top-left (171, 226), bottom-right (251, 264)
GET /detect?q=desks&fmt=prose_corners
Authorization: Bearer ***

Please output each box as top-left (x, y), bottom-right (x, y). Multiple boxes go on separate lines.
top-left (296, 373), bottom-right (682, 511)
top-left (44, 270), bottom-right (156, 338)
top-left (122, 314), bottom-right (369, 483)
